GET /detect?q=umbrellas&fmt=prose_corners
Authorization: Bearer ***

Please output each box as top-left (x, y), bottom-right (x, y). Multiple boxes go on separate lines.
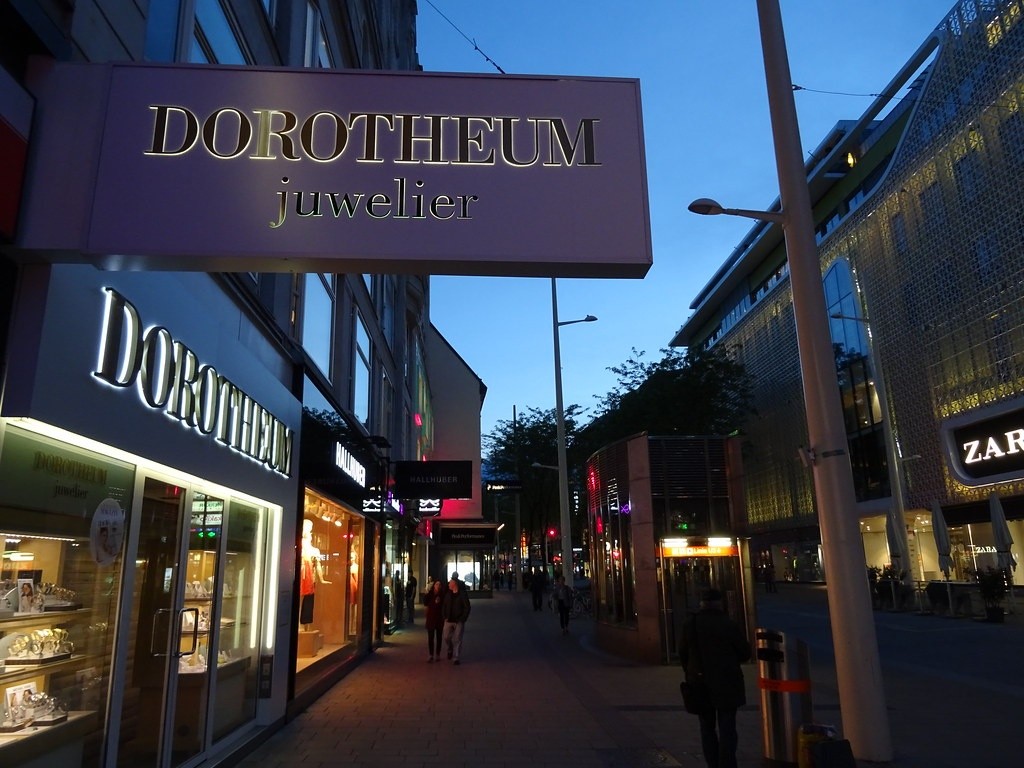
top-left (884, 508), bottom-right (904, 607)
top-left (931, 498), bottom-right (956, 613)
top-left (990, 490), bottom-right (1018, 615)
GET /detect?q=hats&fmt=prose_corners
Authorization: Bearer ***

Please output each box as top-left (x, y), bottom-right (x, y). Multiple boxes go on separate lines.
top-left (702, 589), bottom-right (720, 602)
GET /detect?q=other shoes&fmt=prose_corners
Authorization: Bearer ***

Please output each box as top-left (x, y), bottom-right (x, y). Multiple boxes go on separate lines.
top-left (436, 654), bottom-right (439, 663)
top-left (454, 658), bottom-right (459, 665)
top-left (428, 656), bottom-right (433, 663)
top-left (561, 629), bottom-right (569, 636)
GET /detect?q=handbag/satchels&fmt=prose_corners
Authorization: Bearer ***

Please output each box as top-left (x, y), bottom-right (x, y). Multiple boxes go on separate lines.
top-left (681, 680), bottom-right (697, 714)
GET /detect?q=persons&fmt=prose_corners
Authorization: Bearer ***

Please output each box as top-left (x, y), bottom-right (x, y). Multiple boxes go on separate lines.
top-left (349, 551), bottom-right (359, 634)
top-left (10, 688), bottom-right (33, 707)
top-left (553, 577), bottom-right (574, 634)
top-left (393, 570), bottom-right (404, 624)
top-left (302, 520), bottom-right (332, 630)
top-left (406, 568), bottom-right (417, 624)
top-left (493, 571), bottom-right (514, 592)
top-left (523, 570), bottom-right (543, 611)
top-left (764, 564), bottom-right (777, 595)
top-left (20, 583), bottom-right (34, 612)
top-left (681, 589), bottom-right (747, 768)
top-left (424, 575), bottom-right (472, 665)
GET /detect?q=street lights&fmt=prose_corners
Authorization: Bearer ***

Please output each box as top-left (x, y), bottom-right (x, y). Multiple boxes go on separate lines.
top-left (687, 198), bottom-right (892, 767)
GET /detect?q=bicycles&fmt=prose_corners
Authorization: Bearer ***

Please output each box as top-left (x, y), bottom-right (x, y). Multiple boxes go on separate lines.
top-left (531, 315), bottom-right (599, 593)
top-left (547, 588), bottom-right (594, 619)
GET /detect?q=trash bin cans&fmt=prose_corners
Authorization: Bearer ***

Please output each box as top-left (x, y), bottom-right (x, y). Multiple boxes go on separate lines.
top-left (755, 626), bottom-right (814, 768)
